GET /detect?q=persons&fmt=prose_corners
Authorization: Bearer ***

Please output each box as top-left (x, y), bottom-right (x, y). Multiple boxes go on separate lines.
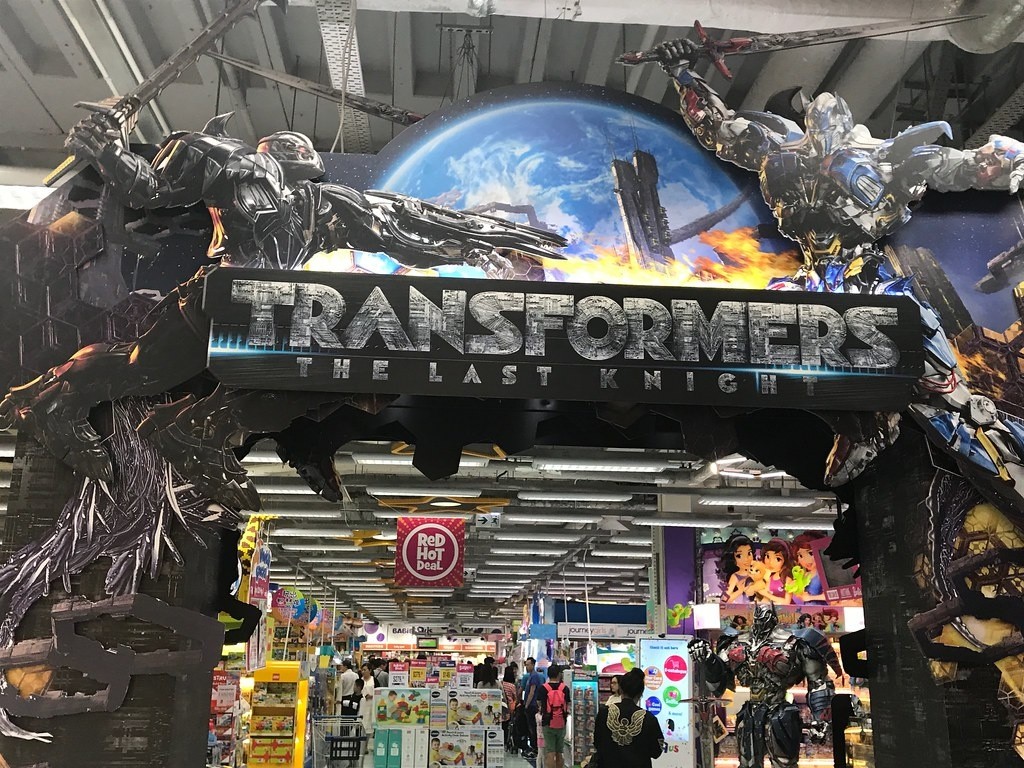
top-left (338, 654), bottom-right (427, 754)
top-left (386, 690), bottom-right (399, 718)
top-left (448, 698), bottom-right (467, 725)
top-left (467, 657), bottom-right (573, 768)
top-left (605, 675), bottom-right (622, 704)
top-left (431, 738), bottom-right (450, 765)
top-left (582, 667), bottom-right (664, 768)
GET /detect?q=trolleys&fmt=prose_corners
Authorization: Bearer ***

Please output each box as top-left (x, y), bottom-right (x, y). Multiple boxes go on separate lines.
top-left (310, 714), bottom-right (370, 768)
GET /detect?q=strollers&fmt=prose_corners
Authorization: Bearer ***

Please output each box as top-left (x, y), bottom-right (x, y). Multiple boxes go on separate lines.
top-left (505, 700), bottom-right (528, 755)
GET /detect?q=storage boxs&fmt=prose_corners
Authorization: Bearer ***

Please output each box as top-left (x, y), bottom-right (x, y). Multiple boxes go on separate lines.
top-left (372, 687), bottom-right (504, 768)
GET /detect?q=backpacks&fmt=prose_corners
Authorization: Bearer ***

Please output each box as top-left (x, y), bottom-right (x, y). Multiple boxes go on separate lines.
top-left (542, 682), bottom-right (567, 729)
top-left (374, 670), bottom-right (382, 687)
